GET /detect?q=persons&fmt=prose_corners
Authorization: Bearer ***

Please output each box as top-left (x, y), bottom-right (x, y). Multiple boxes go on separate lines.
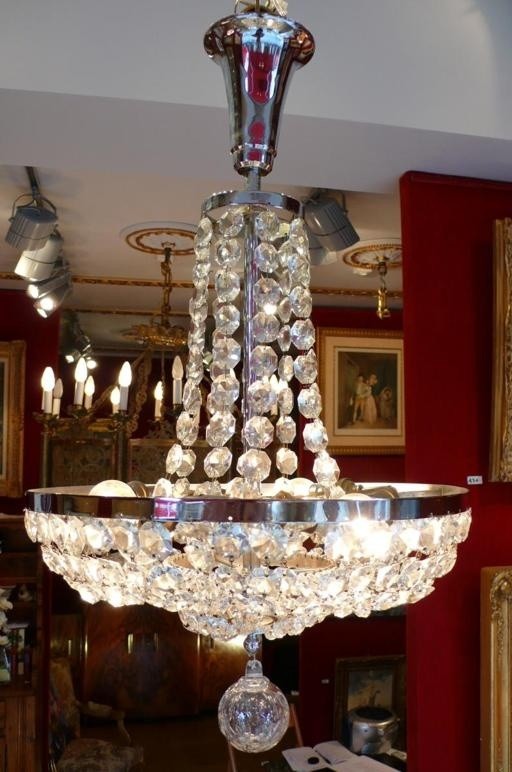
top-left (378, 385), bottom-right (393, 419)
top-left (349, 375), bottom-right (371, 422)
top-left (366, 372), bottom-right (382, 418)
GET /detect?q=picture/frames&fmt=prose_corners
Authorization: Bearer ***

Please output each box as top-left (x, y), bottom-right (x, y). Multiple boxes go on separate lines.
top-left (477, 563), bottom-right (511, 770)
top-left (40, 417), bottom-right (126, 488)
top-left (312, 322), bottom-right (410, 459)
top-left (330, 651), bottom-right (408, 744)
top-left (128, 436), bottom-right (233, 486)
top-left (485, 213), bottom-right (510, 490)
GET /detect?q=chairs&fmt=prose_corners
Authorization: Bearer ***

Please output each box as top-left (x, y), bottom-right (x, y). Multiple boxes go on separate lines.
top-left (221, 700), bottom-right (307, 771)
top-left (46, 653), bottom-right (143, 772)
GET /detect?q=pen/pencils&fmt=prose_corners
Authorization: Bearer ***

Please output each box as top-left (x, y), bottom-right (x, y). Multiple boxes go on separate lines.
top-left (315, 750), bottom-right (332, 764)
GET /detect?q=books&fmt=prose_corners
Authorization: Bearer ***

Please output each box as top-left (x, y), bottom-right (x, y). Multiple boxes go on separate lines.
top-left (280, 739), bottom-right (359, 772)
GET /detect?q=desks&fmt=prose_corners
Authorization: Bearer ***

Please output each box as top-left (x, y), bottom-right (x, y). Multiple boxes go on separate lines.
top-left (261, 746), bottom-right (408, 771)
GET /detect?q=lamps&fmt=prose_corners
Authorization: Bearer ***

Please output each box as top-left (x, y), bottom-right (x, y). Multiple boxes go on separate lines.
top-left (65, 336), bottom-right (98, 369)
top-left (21, 1), bottom-right (475, 760)
top-left (35, 229), bottom-right (198, 430)
top-left (4, 179), bottom-right (78, 322)
